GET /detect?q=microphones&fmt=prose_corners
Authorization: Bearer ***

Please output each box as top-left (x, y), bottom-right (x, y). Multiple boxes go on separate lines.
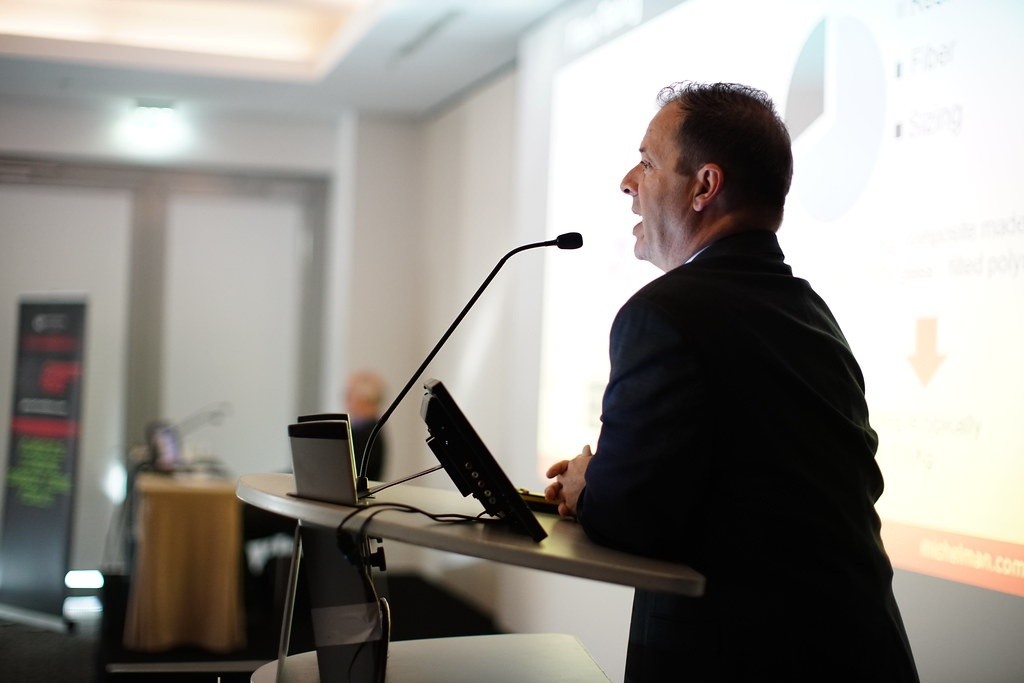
top-left (354, 231), bottom-right (584, 494)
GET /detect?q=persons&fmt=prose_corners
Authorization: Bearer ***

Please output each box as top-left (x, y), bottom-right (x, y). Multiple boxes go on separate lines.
top-left (543, 78), bottom-right (920, 683)
top-left (115, 420), bottom-right (216, 579)
top-left (240, 370), bottom-right (387, 610)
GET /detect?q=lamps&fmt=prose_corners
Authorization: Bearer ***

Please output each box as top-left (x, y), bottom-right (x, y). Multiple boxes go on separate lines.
top-left (116, 97), bottom-right (187, 159)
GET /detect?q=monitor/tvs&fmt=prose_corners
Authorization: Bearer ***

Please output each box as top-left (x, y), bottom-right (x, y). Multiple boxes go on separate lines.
top-left (418, 380), bottom-right (548, 543)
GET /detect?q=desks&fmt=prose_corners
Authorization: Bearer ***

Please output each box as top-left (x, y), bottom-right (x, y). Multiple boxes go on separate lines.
top-left (122, 469), bottom-right (246, 658)
